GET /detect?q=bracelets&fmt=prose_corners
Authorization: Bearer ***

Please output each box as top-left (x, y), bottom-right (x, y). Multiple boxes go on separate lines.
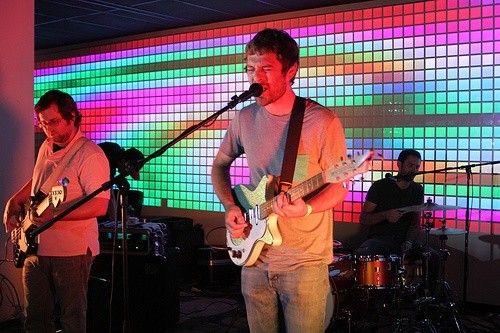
top-left (53, 208), bottom-right (60, 218)
top-left (301, 204), bottom-right (312, 219)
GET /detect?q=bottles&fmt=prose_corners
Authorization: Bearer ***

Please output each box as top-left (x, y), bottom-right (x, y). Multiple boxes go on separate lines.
top-left (154, 226), bottom-right (164, 257)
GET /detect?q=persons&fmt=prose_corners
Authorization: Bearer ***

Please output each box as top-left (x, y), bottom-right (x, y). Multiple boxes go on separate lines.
top-left (354, 148), bottom-right (454, 299)
top-left (98, 142), bottom-right (146, 222)
top-left (210, 27), bottom-right (353, 333)
top-left (2, 89), bottom-right (114, 333)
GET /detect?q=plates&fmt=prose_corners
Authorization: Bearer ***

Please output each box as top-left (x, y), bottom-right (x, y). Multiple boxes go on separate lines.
top-left (126, 222), bottom-right (142, 226)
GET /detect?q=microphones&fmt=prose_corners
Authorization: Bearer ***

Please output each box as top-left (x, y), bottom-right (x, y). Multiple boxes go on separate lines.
top-left (226, 83), bottom-right (263, 110)
top-left (385, 173), bottom-right (400, 182)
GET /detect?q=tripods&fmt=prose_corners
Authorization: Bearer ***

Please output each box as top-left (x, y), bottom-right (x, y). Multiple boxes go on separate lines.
top-left (380, 213), bottom-right (468, 333)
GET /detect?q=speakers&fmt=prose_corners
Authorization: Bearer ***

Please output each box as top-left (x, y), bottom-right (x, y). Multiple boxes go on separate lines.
top-left (148, 216), bottom-right (195, 281)
top-left (88, 253), bottom-right (180, 333)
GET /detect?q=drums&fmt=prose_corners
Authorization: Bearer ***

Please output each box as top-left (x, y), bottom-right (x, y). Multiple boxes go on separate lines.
top-left (358, 252), bottom-right (404, 289)
top-left (332, 238), bottom-right (342, 247)
top-left (330, 255), bottom-right (356, 288)
top-left (327, 278), bottom-right (339, 330)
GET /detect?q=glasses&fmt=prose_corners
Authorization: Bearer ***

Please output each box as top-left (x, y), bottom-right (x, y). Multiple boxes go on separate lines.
top-left (38, 117), bottom-right (63, 128)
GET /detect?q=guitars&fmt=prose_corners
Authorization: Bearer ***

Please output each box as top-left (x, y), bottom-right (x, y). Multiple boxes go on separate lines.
top-left (11, 175), bottom-right (69, 268)
top-left (225, 148), bottom-right (377, 266)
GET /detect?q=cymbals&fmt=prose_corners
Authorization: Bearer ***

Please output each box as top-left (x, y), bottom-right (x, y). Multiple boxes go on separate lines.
top-left (414, 227), bottom-right (466, 235)
top-left (396, 203), bottom-right (459, 212)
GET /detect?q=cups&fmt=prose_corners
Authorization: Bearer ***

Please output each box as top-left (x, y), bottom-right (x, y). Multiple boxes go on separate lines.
top-left (128, 217), bottom-right (139, 224)
